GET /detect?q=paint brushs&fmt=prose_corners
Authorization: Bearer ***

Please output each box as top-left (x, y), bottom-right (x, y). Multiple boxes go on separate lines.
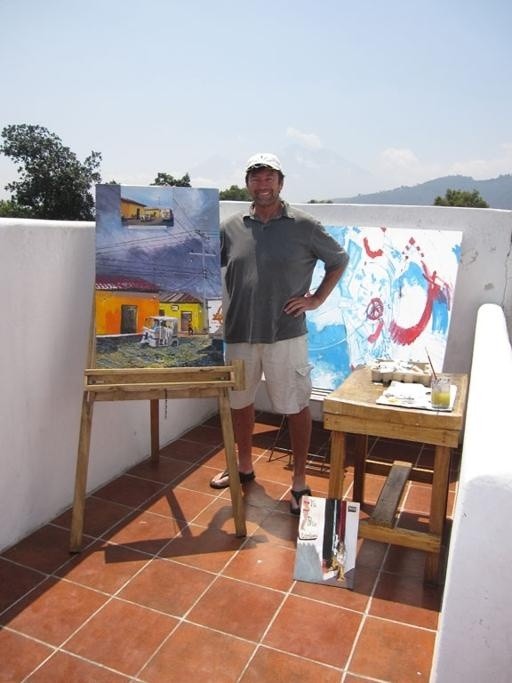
top-left (425, 347), bottom-right (437, 380)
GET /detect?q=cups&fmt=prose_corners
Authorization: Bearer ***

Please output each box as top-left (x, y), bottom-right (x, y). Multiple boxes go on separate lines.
top-left (430, 376), bottom-right (450, 409)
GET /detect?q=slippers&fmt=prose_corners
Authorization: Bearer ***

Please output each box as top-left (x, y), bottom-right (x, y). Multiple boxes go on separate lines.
top-left (210, 468), bottom-right (255, 490)
top-left (290, 485), bottom-right (311, 514)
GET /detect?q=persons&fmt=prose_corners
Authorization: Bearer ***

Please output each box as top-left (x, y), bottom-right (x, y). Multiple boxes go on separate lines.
top-left (326, 539), bottom-right (351, 581)
top-left (300, 496), bottom-right (315, 539)
top-left (152, 318), bottom-right (159, 331)
top-left (187, 318), bottom-right (194, 335)
top-left (206, 151), bottom-right (352, 517)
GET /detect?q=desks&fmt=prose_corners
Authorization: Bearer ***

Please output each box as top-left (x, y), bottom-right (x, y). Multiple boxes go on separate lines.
top-left (320, 362), bottom-right (468, 591)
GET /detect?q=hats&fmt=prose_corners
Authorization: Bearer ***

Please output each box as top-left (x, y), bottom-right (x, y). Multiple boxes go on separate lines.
top-left (246, 153), bottom-right (285, 176)
